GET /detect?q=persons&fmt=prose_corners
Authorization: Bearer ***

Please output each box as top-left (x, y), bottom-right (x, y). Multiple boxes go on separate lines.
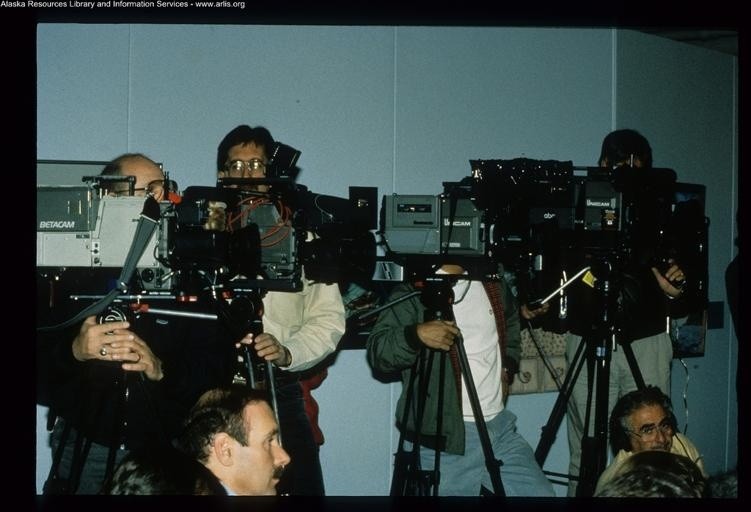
top-left (217, 125), bottom-right (340, 496)
top-left (592, 451), bottom-right (738, 499)
top-left (176, 388), bottom-right (291, 496)
top-left (36, 153), bottom-right (223, 495)
top-left (105, 445), bottom-right (191, 495)
top-left (594, 385), bottom-right (703, 492)
top-left (366, 263), bottom-right (556, 496)
top-left (566, 129), bottom-right (686, 497)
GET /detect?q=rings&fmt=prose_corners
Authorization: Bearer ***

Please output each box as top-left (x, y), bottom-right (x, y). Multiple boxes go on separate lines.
top-left (99, 345), bottom-right (107, 356)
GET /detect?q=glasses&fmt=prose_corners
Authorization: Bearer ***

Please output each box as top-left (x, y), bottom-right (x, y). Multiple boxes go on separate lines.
top-left (627, 425), bottom-right (674, 441)
top-left (223, 159), bottom-right (265, 178)
top-left (117, 180), bottom-right (177, 200)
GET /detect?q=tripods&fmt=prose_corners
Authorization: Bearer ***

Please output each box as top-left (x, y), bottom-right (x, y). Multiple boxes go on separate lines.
top-left (66, 360), bottom-right (173, 495)
top-left (534, 278), bottom-right (647, 497)
top-left (389, 281), bottom-right (506, 497)
